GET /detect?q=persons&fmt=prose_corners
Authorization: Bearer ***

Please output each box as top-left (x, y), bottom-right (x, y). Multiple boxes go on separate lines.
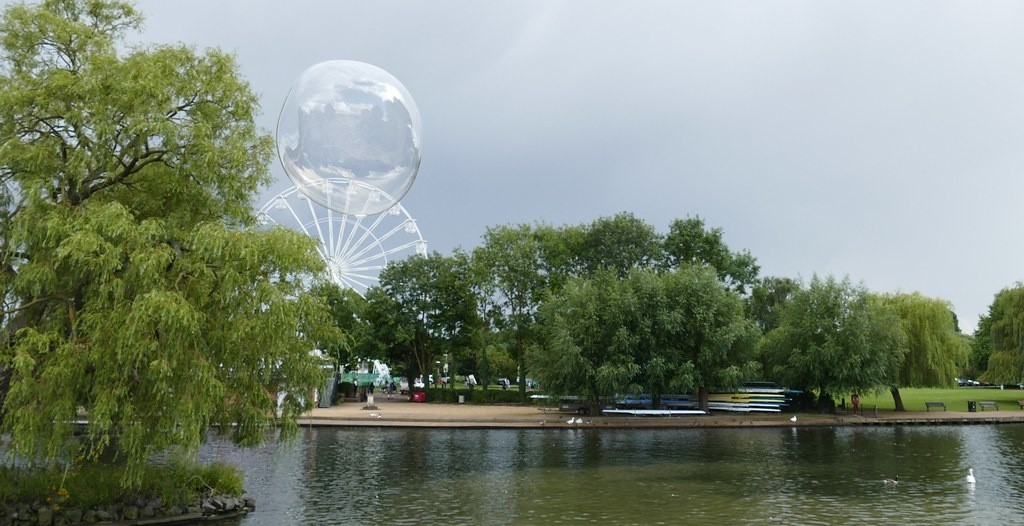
top-left (369, 379), bottom-right (397, 394)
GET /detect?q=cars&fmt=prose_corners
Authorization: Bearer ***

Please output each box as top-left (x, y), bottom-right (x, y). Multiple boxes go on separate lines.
top-left (955, 377), bottom-right (999, 387)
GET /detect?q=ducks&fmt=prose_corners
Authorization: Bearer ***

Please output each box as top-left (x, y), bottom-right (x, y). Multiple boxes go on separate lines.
top-left (369, 412), bottom-right (382, 419)
top-left (790, 415), bottom-right (798, 423)
top-left (539, 415), bottom-right (594, 426)
top-left (883, 475), bottom-right (898, 485)
top-left (967, 468), bottom-right (976, 483)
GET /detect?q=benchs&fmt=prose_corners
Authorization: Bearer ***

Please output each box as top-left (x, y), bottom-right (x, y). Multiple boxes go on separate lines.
top-left (979, 401), bottom-right (999, 411)
top-left (925, 402), bottom-right (947, 412)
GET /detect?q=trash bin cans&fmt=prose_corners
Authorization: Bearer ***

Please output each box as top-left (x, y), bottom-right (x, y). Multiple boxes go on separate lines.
top-left (967, 400), bottom-right (976, 412)
